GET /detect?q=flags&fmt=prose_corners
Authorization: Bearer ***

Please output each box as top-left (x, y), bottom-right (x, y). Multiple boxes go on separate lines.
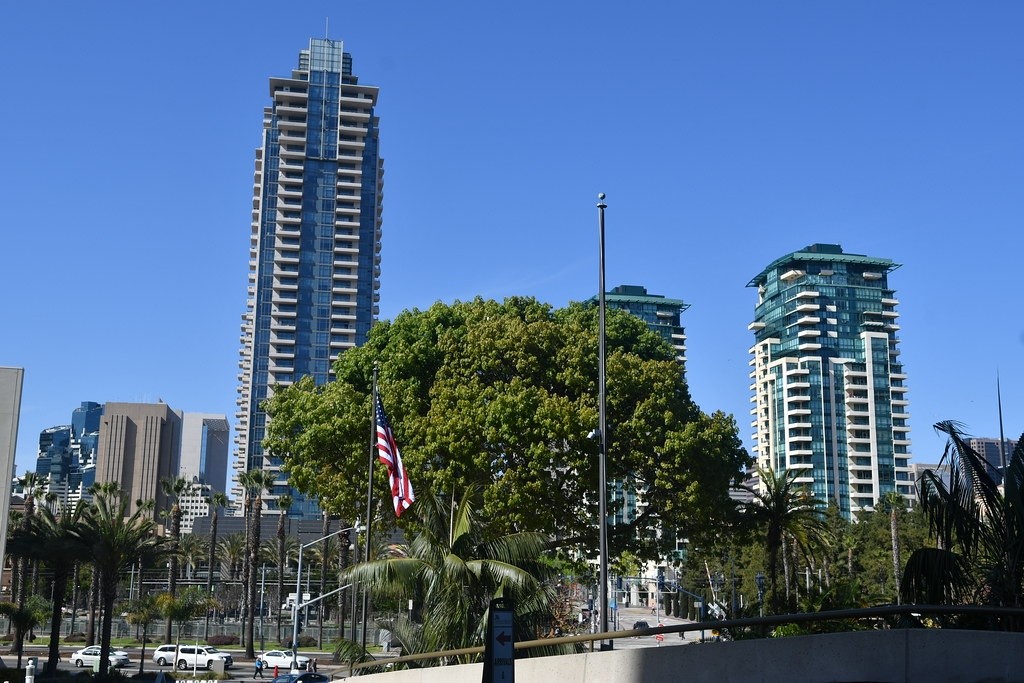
top-left (375, 384), bottom-right (416, 518)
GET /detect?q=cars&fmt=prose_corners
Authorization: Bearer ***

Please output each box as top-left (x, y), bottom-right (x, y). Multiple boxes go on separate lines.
top-left (271, 673), bottom-right (330, 683)
top-left (72, 646), bottom-right (128, 660)
top-left (256, 650), bottom-right (310, 669)
top-left (153, 645), bottom-right (183, 666)
top-left (69, 650), bottom-right (129, 668)
top-left (632, 620), bottom-right (649, 631)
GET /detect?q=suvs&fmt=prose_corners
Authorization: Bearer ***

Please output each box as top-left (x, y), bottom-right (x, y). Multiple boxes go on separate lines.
top-left (173, 645), bottom-right (234, 671)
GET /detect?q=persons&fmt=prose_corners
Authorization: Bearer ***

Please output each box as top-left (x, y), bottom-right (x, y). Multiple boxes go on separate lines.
top-left (253, 657), bottom-right (264, 679)
top-left (313, 658), bottom-right (319, 673)
top-left (306, 659), bottom-right (313, 673)
top-left (56, 650), bottom-right (61, 669)
